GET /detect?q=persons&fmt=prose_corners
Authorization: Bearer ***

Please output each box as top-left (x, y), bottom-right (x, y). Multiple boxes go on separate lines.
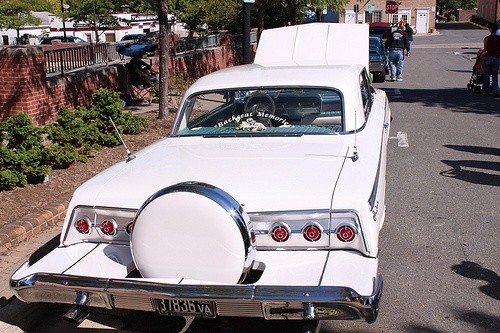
top-left (128, 52), bottom-right (159, 96)
top-left (482, 22), bottom-right (500, 94)
top-left (385, 23), bottom-right (414, 82)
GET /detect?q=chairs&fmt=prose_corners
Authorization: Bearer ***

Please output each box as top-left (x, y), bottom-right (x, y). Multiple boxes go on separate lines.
top-left (301, 114), bottom-right (342, 125)
top-left (214, 116), bottom-right (274, 127)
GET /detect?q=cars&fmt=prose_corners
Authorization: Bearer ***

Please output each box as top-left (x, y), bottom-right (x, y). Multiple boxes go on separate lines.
top-left (115, 34), bottom-right (154, 55)
top-left (41, 36), bottom-right (86, 45)
top-left (369, 22), bottom-right (393, 81)
top-left (8, 21), bottom-right (392, 325)
top-left (390, 22), bottom-right (415, 36)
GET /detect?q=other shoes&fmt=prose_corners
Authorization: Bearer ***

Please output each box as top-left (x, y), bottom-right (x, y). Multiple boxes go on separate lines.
top-left (391, 78), bottom-right (396, 80)
top-left (397, 78), bottom-right (403, 81)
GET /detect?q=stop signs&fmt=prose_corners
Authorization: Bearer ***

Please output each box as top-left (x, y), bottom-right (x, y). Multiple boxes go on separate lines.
top-left (386, 1), bottom-right (399, 14)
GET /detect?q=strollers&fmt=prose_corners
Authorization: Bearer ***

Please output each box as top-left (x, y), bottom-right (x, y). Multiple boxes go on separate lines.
top-left (468, 47), bottom-right (488, 94)
